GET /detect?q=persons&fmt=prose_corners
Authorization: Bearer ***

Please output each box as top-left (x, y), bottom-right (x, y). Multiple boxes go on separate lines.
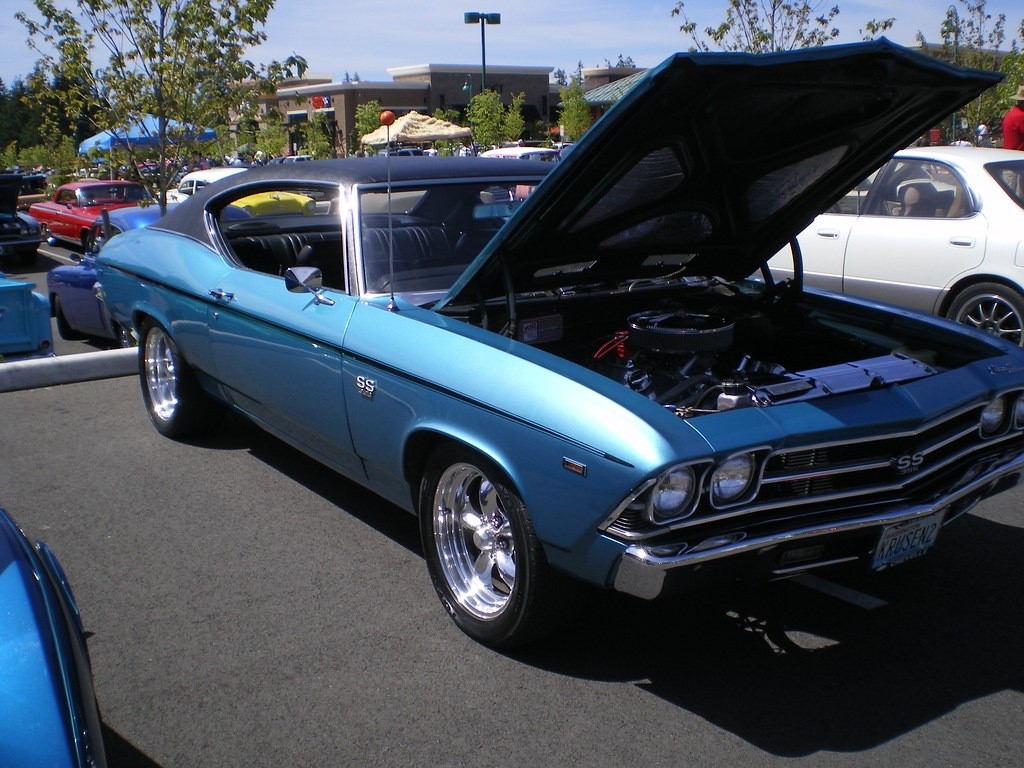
top-left (184, 150), bottom-right (210, 170)
top-left (1001, 84), bottom-right (1024, 152)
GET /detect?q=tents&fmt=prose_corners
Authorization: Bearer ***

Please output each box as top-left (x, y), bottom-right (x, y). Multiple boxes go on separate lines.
top-left (362, 110), bottom-right (471, 150)
top-left (78, 110), bottom-right (216, 171)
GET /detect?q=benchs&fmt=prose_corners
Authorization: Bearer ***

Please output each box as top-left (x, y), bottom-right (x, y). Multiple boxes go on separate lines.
top-left (234, 226), bottom-right (450, 287)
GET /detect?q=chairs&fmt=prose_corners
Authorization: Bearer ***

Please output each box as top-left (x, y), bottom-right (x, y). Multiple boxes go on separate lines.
top-left (898, 183), bottom-right (955, 216)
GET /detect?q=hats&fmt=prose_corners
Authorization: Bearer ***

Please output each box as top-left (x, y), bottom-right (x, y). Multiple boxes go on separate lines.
top-left (1008, 85), bottom-right (1024, 100)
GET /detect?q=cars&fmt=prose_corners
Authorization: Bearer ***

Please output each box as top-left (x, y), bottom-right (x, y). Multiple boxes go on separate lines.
top-left (27, 178), bottom-right (159, 250)
top-left (43, 200), bottom-right (255, 349)
top-left (0, 504), bottom-right (110, 768)
top-left (86, 34), bottom-right (1024, 653)
top-left (0, 171), bottom-right (47, 261)
top-left (5, 142), bottom-right (576, 218)
top-left (746, 146), bottom-right (1024, 348)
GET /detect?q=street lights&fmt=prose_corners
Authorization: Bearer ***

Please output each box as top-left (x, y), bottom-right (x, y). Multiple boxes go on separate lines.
top-left (462, 74), bottom-right (473, 132)
top-left (940, 4), bottom-right (960, 145)
top-left (463, 10), bottom-right (502, 94)
